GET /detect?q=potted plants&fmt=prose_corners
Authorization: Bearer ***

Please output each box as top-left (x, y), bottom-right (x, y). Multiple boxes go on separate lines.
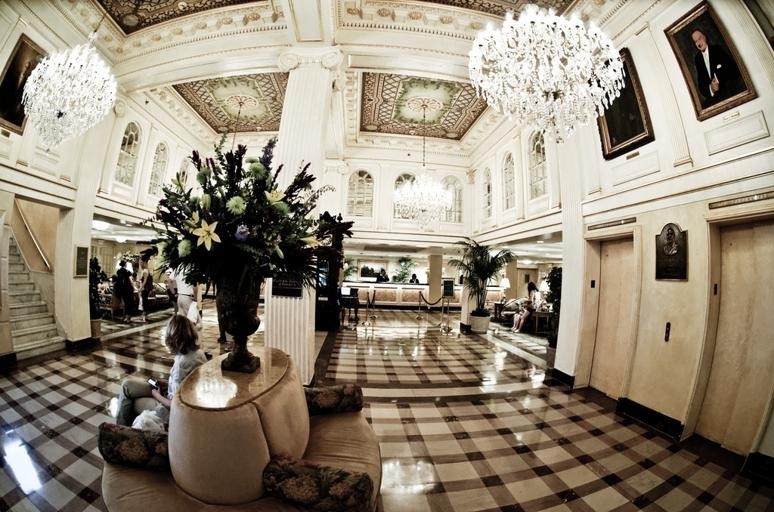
top-left (543, 265), bottom-right (563, 366)
top-left (446, 240), bottom-right (520, 336)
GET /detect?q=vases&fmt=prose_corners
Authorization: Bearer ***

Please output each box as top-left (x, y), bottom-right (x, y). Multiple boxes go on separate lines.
top-left (215, 278), bottom-right (263, 375)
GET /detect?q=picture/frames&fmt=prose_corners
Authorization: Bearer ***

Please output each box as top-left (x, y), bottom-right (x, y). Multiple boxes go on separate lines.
top-left (661, 1), bottom-right (760, 124)
top-left (0, 32), bottom-right (52, 139)
top-left (587, 45), bottom-right (658, 162)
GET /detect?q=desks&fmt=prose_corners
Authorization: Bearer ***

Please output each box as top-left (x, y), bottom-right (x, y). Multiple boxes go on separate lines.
top-left (527, 307), bottom-right (553, 337)
top-left (492, 300), bottom-right (508, 324)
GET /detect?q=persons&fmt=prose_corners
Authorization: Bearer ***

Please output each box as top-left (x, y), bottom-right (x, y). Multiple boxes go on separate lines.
top-left (0, 61), bottom-right (32, 126)
top-left (511, 279), bottom-right (539, 333)
top-left (114, 314), bottom-right (209, 433)
top-left (89, 253), bottom-right (154, 324)
top-left (375, 267), bottom-right (390, 283)
top-left (691, 29), bottom-right (743, 110)
top-left (409, 274), bottom-right (420, 284)
top-left (167, 263), bottom-right (197, 318)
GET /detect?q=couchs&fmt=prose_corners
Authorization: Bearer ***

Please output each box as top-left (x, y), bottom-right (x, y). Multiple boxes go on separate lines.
top-left (94, 280), bottom-right (167, 318)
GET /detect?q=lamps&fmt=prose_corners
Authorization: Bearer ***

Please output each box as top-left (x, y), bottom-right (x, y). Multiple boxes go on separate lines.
top-left (469, 2), bottom-right (626, 144)
top-left (21, 0), bottom-right (118, 152)
top-left (391, 101), bottom-right (454, 216)
top-left (498, 277), bottom-right (510, 302)
top-left (539, 281), bottom-right (551, 298)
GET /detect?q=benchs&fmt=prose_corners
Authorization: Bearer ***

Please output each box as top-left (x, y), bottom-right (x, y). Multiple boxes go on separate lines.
top-left (97, 356), bottom-right (383, 512)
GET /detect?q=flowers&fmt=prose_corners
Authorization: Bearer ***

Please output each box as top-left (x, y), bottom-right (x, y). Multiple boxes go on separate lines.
top-left (133, 121), bottom-right (353, 288)
